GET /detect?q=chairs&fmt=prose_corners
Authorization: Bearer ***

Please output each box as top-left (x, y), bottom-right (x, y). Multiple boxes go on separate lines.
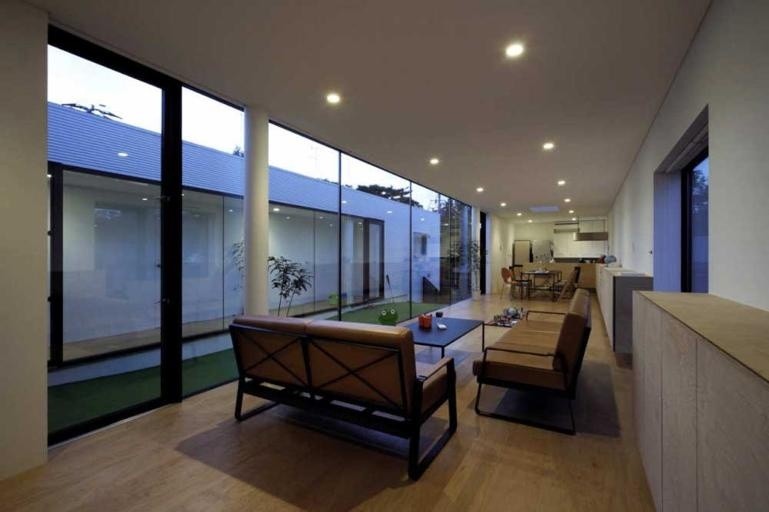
top-left (501, 265), bottom-right (578, 301)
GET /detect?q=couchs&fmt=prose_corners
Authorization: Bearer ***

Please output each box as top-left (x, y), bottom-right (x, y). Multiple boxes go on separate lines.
top-left (229, 315), bottom-right (457, 481)
top-left (474, 289), bottom-right (591, 436)
